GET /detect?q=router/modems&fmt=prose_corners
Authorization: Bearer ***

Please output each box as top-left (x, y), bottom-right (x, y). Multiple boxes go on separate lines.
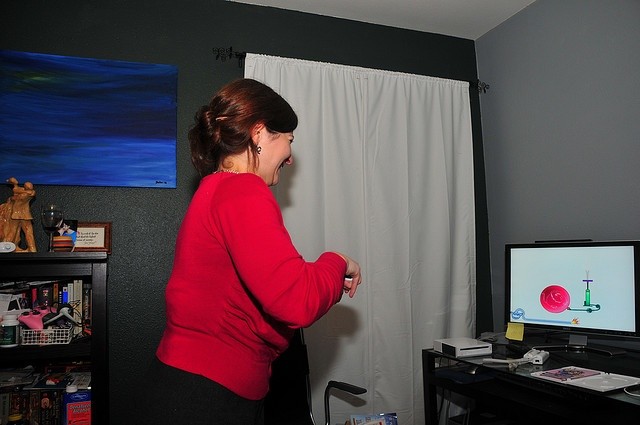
top-left (432, 336), bottom-right (494, 358)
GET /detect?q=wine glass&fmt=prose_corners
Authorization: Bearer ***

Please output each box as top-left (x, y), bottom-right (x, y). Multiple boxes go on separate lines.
top-left (41, 204), bottom-right (63, 253)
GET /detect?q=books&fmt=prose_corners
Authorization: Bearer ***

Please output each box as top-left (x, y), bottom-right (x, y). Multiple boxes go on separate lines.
top-left (0, 371), bottom-right (68, 425)
top-left (15, 280), bottom-right (91, 336)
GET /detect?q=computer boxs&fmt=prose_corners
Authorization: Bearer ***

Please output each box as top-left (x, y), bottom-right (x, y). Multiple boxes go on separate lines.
top-left (447, 410), bottom-right (523, 424)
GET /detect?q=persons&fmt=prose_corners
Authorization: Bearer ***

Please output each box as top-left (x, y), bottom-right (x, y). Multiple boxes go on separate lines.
top-left (63, 223), bottom-right (76, 252)
top-left (0, 177), bottom-right (37, 252)
top-left (154, 78), bottom-right (362, 424)
top-left (55, 228), bottom-right (65, 235)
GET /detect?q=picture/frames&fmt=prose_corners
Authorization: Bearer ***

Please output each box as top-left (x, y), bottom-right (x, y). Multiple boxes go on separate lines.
top-left (73, 222), bottom-right (112, 254)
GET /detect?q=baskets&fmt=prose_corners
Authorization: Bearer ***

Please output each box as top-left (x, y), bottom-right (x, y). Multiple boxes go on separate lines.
top-left (20, 324), bottom-right (74, 345)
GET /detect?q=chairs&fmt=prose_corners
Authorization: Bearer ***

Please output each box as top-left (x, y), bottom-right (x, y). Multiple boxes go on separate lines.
top-left (262, 326), bottom-right (367, 425)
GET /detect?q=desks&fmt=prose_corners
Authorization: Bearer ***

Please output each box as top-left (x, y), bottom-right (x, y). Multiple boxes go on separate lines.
top-left (422, 338), bottom-right (640, 425)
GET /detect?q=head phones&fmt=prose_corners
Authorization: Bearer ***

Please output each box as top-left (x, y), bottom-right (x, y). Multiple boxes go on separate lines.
top-left (41, 301), bottom-right (72, 325)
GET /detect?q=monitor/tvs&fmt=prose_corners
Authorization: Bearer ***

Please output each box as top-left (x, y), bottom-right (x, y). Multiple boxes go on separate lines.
top-left (503, 241), bottom-right (638, 357)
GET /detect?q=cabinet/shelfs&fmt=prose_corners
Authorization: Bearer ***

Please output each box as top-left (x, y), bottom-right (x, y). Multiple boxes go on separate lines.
top-left (0, 252), bottom-right (111, 425)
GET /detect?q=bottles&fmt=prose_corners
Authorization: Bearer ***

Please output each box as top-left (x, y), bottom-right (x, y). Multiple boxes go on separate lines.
top-left (1, 314), bottom-right (20, 347)
top-left (8, 414), bottom-right (23, 424)
